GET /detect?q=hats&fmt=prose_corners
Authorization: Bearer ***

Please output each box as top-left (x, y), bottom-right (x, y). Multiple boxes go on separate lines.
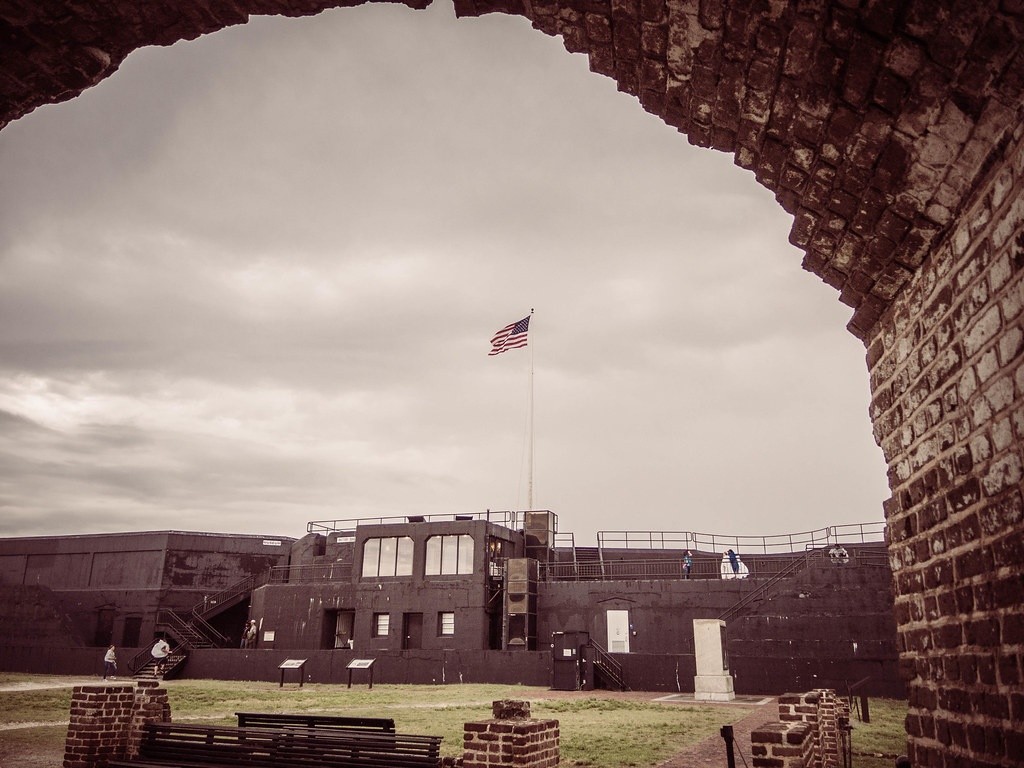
top-left (251, 619), bottom-right (256, 623)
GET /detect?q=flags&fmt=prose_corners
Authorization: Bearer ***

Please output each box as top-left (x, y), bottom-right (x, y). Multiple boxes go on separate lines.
top-left (487, 315), bottom-right (531, 355)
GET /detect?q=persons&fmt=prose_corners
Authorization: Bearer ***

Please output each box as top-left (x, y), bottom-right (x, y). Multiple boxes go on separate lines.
top-left (240, 619), bottom-right (257, 648)
top-left (724, 549), bottom-right (739, 573)
top-left (152, 638), bottom-right (172, 677)
top-left (683, 551), bottom-right (693, 579)
top-left (103, 645), bottom-right (117, 682)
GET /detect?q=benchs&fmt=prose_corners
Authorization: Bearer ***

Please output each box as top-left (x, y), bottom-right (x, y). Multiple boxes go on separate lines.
top-left (108, 701), bottom-right (445, 768)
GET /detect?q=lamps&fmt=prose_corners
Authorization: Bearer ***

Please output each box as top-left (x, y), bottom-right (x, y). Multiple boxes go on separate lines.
top-left (456, 515), bottom-right (473, 521)
top-left (407, 516), bottom-right (427, 523)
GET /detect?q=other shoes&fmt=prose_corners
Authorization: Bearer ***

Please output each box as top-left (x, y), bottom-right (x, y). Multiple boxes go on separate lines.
top-left (154, 674), bottom-right (156, 676)
top-left (110, 676), bottom-right (116, 679)
top-left (103, 679), bottom-right (108, 682)
top-left (161, 671), bottom-right (164, 674)
